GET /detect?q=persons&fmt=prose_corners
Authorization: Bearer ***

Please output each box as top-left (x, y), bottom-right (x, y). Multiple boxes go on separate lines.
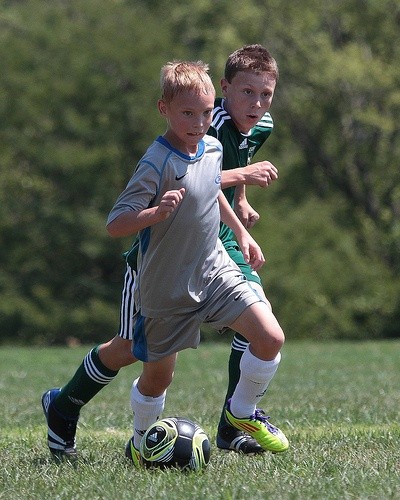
top-left (42, 46), bottom-right (279, 461)
top-left (106, 60), bottom-right (290, 469)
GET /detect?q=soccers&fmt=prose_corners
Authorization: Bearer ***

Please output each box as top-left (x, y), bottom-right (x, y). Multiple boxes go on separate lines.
top-left (138, 415), bottom-right (211, 474)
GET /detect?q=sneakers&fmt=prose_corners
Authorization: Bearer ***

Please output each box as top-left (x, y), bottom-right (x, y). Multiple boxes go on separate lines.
top-left (217, 407), bottom-right (265, 452)
top-left (126, 437), bottom-right (142, 471)
top-left (224, 399), bottom-right (290, 452)
top-left (41, 389), bottom-right (80, 468)
